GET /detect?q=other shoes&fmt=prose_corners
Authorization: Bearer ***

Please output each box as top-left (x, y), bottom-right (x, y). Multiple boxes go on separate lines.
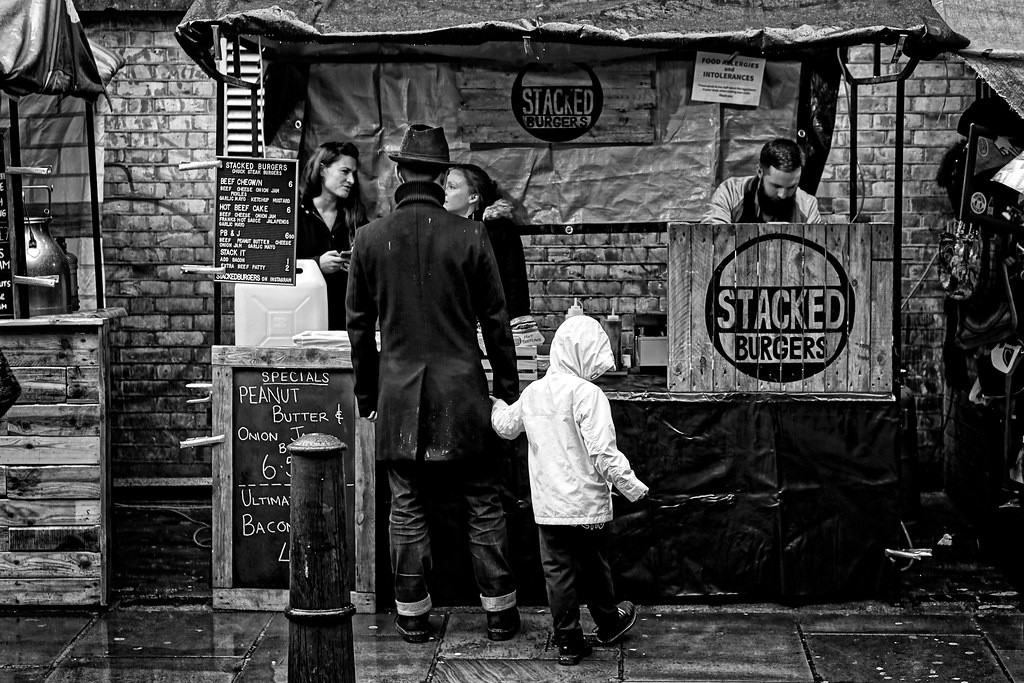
top-left (486, 606), bottom-right (521, 641)
top-left (394, 610), bottom-right (430, 642)
top-left (596, 601), bottom-right (638, 645)
top-left (558, 643), bottom-right (593, 665)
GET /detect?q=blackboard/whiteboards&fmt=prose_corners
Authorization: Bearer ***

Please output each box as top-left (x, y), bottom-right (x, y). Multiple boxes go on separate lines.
top-left (212, 345), bottom-right (376, 613)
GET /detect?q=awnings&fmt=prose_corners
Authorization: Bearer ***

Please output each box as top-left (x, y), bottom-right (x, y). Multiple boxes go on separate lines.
top-left (174, 0), bottom-right (970, 400)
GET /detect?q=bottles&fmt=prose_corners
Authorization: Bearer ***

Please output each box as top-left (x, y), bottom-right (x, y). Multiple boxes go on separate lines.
top-left (604, 306), bottom-right (622, 370)
top-left (565, 297), bottom-right (584, 321)
top-left (57, 238), bottom-right (80, 311)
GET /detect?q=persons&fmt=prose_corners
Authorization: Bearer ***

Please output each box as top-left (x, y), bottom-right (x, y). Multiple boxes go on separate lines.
top-left (299, 124), bottom-right (531, 643)
top-left (489, 314), bottom-right (649, 665)
top-left (700, 138), bottom-right (822, 224)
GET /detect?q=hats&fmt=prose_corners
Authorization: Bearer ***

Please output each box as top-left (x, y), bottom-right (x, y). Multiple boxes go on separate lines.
top-left (388, 123), bottom-right (460, 168)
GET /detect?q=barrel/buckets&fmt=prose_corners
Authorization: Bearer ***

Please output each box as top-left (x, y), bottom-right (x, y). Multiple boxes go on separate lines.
top-left (233, 259), bottom-right (328, 349)
